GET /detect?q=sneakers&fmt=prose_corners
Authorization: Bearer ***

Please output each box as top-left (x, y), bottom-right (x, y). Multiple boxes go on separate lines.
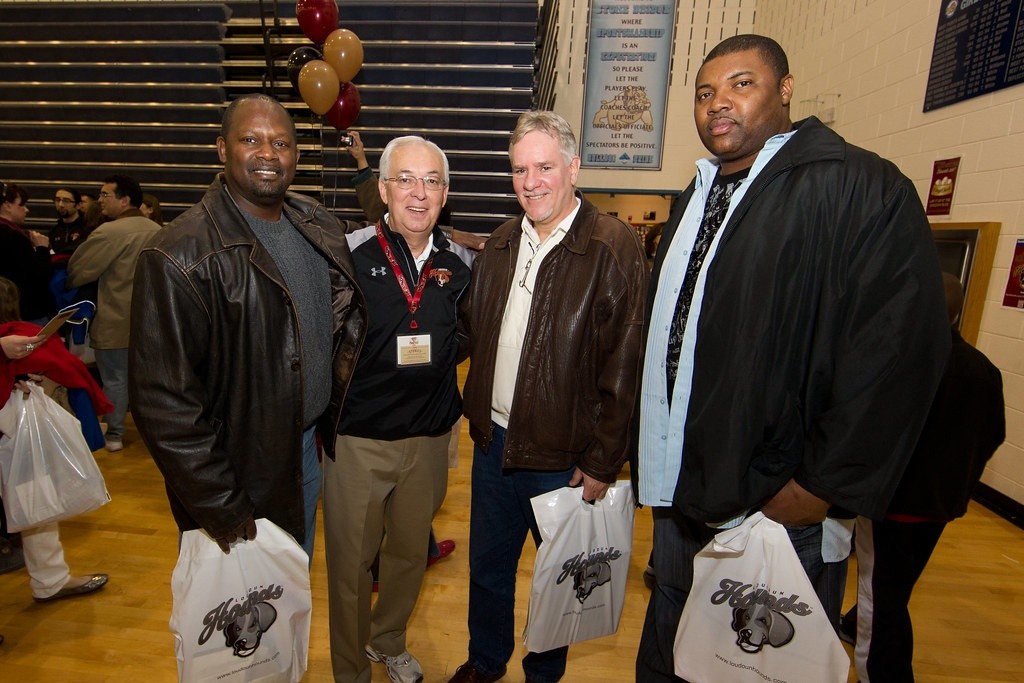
top-left (365, 644), bottom-right (423, 683)
top-left (372, 582), bottom-right (379, 591)
top-left (427, 540), bottom-right (455, 566)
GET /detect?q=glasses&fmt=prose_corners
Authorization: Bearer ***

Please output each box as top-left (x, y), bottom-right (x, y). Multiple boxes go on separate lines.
top-left (98, 194), bottom-right (124, 200)
top-left (385, 175), bottom-right (446, 191)
top-left (54, 197), bottom-right (75, 204)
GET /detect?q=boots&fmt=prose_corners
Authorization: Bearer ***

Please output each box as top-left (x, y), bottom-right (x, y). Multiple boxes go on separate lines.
top-left (0, 534), bottom-right (25, 574)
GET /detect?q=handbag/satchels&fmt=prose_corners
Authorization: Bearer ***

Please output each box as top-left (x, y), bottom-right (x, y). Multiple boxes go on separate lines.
top-left (169, 517), bottom-right (311, 683)
top-left (0, 380), bottom-right (112, 533)
top-left (522, 480), bottom-right (636, 654)
top-left (673, 511), bottom-right (851, 683)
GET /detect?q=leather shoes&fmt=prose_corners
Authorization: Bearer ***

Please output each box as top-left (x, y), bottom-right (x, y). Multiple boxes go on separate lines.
top-left (447, 661), bottom-right (506, 683)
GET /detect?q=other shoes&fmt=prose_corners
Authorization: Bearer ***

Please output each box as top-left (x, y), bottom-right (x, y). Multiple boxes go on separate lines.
top-left (647, 557), bottom-right (655, 577)
top-left (104, 439), bottom-right (124, 452)
top-left (839, 613), bottom-right (857, 646)
top-left (32, 574), bottom-right (109, 602)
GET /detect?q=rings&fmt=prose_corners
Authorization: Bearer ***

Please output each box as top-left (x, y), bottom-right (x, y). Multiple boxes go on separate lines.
top-left (27, 344), bottom-right (34, 352)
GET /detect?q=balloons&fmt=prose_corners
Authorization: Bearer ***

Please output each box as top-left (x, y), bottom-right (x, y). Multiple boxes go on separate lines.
top-left (298, 60), bottom-right (340, 119)
top-left (324, 28), bottom-right (363, 82)
top-left (296, 0), bottom-right (340, 45)
top-left (287, 47), bottom-right (323, 96)
top-left (324, 81), bottom-right (360, 132)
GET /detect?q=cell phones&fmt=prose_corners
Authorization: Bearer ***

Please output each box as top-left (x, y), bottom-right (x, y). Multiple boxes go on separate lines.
top-left (338, 135), bottom-right (353, 147)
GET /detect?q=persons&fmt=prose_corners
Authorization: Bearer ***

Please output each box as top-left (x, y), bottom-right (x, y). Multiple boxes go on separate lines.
top-left (628, 34), bottom-right (944, 683)
top-left (447, 112), bottom-right (652, 683)
top-left (130, 94), bottom-right (488, 567)
top-left (0, 182), bottom-right (163, 452)
top-left (342, 131), bottom-right (386, 222)
top-left (841, 274), bottom-right (1006, 683)
top-left (371, 525), bottom-right (455, 592)
top-left (0, 334), bottom-right (109, 645)
top-left (318, 136), bottom-right (472, 683)
